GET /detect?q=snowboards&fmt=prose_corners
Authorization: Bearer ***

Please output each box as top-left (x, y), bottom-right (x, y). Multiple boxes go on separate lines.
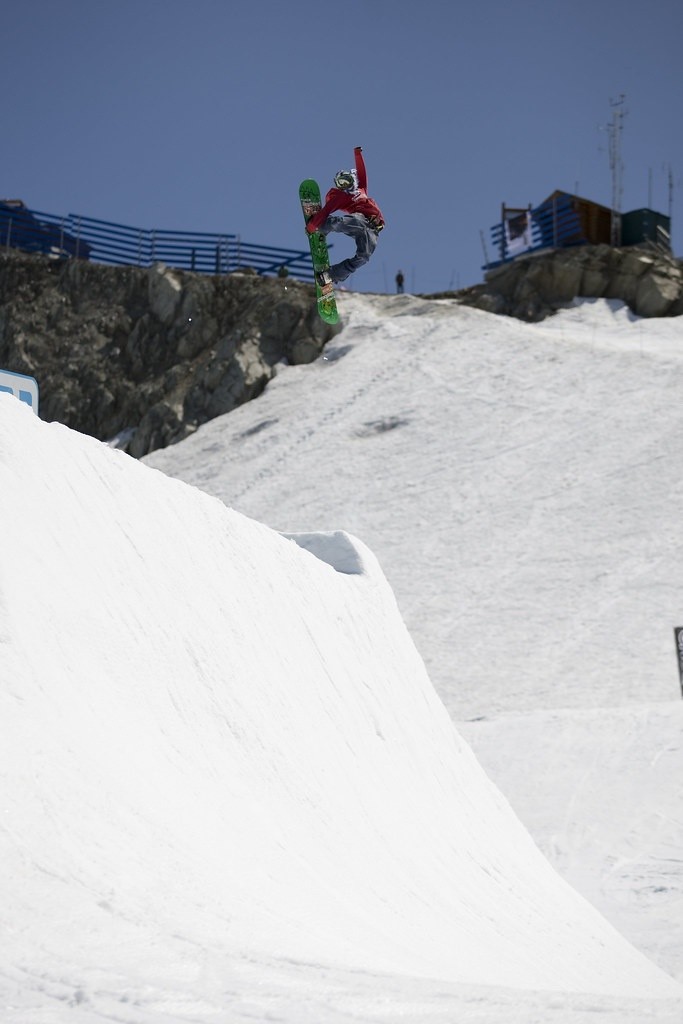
top-left (294, 177), bottom-right (342, 328)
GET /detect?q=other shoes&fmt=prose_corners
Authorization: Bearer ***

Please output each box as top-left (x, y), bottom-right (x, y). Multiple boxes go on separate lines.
top-left (318, 271), bottom-right (333, 285)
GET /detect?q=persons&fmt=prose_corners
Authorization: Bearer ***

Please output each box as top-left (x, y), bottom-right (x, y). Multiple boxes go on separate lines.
top-left (396, 270), bottom-right (404, 293)
top-left (303, 147), bottom-right (385, 287)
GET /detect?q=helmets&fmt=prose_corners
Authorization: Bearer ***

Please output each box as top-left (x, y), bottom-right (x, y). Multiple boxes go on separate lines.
top-left (335, 170), bottom-right (357, 192)
